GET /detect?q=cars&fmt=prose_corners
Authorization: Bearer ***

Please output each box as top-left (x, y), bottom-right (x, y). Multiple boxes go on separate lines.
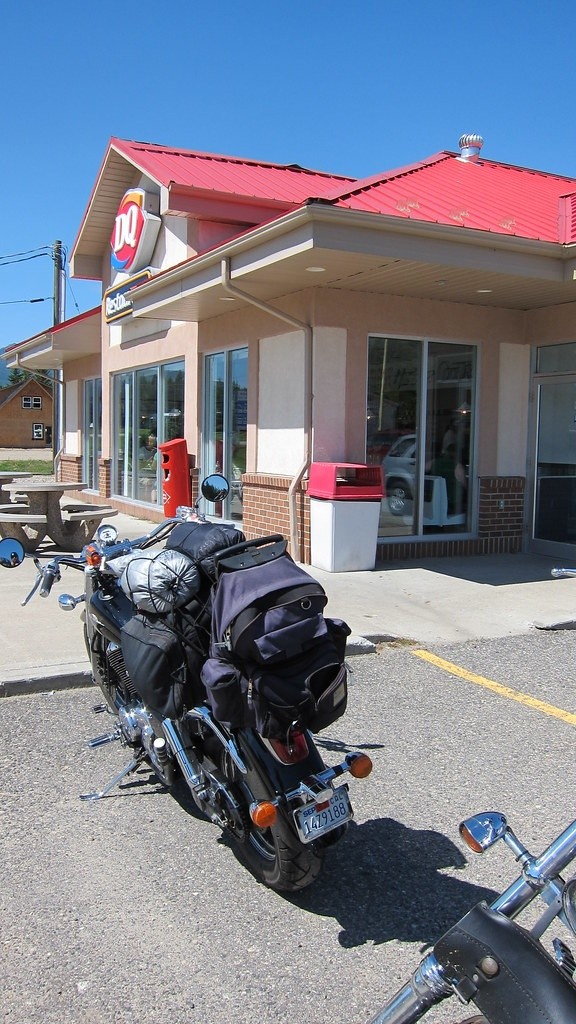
top-left (366, 428), bottom-right (465, 518)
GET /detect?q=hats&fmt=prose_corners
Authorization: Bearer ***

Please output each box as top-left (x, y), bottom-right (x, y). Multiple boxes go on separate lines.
top-left (11, 552), bottom-right (18, 559)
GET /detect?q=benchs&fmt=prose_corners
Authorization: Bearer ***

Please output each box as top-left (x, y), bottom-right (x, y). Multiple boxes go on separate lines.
top-left (0, 491), bottom-right (118, 554)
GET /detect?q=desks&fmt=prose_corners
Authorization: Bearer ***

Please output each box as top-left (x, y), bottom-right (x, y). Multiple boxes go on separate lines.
top-left (0, 472), bottom-right (87, 554)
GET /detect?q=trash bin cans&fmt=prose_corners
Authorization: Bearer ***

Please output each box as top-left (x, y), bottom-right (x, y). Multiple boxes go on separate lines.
top-left (157, 438), bottom-right (191, 517)
top-left (305, 461), bottom-right (386, 573)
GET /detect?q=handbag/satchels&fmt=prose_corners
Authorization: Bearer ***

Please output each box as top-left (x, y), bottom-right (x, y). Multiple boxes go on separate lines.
top-left (118, 599), bottom-right (212, 721)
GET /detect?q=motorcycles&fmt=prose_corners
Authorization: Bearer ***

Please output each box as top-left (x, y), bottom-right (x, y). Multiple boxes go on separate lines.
top-left (365, 809), bottom-right (576, 1024)
top-left (1, 471), bottom-right (385, 895)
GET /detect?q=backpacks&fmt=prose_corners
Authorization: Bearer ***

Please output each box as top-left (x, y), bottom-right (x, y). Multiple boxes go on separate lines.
top-left (200, 554), bottom-right (354, 740)
